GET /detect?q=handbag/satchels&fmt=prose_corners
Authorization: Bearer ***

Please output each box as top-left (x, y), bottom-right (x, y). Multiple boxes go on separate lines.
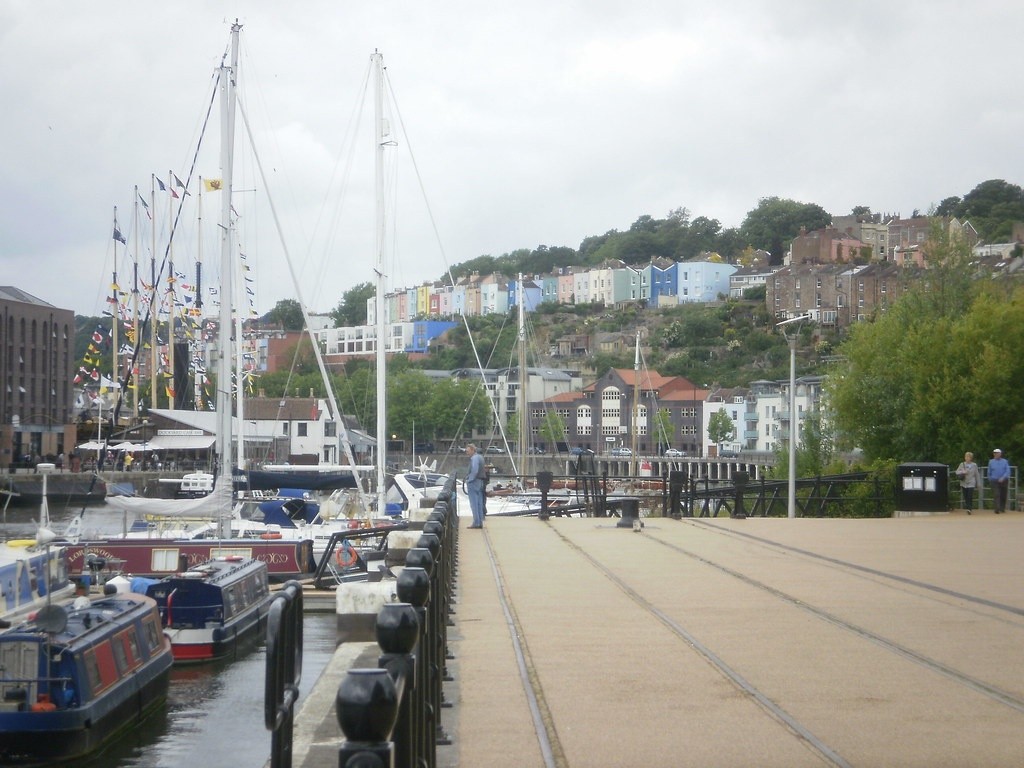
top-left (958, 462), bottom-right (965, 481)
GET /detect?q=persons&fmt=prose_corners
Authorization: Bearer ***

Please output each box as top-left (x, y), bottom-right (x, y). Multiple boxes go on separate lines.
top-left (465, 443), bottom-right (485, 530)
top-left (23, 450), bottom-right (139, 473)
top-left (987, 449), bottom-right (1011, 513)
top-left (955, 452), bottom-right (980, 516)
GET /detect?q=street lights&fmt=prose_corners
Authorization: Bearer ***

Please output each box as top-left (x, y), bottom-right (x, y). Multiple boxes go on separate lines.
top-left (776, 317), bottom-right (802, 517)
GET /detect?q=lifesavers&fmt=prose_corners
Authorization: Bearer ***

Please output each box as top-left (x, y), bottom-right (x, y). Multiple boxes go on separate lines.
top-left (261, 533), bottom-right (283, 540)
top-left (335, 545), bottom-right (358, 567)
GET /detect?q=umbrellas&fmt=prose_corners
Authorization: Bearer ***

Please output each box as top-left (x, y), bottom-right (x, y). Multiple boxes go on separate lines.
top-left (78, 442), bottom-right (164, 453)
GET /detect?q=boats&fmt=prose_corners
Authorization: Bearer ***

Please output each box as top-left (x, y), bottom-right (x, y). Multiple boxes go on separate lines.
top-left (0, 462), bottom-right (172, 768)
top-left (160, 462), bottom-right (553, 518)
top-left (63, 543), bottom-right (276, 664)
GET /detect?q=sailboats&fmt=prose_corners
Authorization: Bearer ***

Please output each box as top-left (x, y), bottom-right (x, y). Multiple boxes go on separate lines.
top-left (484, 272), bottom-right (698, 506)
top-left (94, 16), bottom-right (399, 612)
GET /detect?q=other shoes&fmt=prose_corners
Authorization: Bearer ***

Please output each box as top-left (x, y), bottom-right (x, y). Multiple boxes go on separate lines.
top-left (467, 526), bottom-right (482, 529)
top-left (995, 509), bottom-right (999, 514)
top-left (967, 509), bottom-right (971, 515)
top-left (1000, 508), bottom-right (1004, 512)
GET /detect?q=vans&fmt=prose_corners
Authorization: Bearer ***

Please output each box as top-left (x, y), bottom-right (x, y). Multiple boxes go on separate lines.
top-left (719, 449), bottom-right (738, 458)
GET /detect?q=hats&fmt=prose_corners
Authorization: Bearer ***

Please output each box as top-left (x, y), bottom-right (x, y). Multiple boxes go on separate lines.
top-left (993, 448), bottom-right (1001, 453)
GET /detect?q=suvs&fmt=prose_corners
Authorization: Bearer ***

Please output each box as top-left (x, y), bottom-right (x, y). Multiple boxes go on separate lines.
top-left (414, 443), bottom-right (434, 453)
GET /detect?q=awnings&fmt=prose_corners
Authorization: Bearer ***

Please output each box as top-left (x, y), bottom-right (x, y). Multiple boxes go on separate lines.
top-left (147, 436), bottom-right (216, 449)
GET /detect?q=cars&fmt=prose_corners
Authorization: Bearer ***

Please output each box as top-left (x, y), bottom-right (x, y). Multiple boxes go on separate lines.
top-left (611, 448), bottom-right (620, 456)
top-left (450, 446), bottom-right (465, 454)
top-left (569, 447), bottom-right (583, 455)
top-left (619, 448), bottom-right (636, 456)
top-left (486, 446), bottom-right (503, 453)
top-left (665, 448), bottom-right (687, 457)
top-left (529, 447), bottom-right (545, 454)
